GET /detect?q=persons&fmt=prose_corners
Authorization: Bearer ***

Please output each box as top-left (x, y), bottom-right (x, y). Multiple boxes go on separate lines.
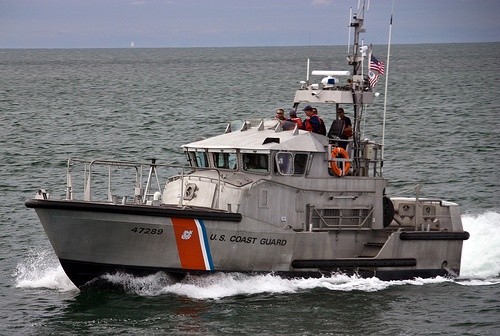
top-left (337, 108), bottom-right (352, 169)
top-left (302, 106), bottom-right (326, 136)
top-left (275, 109), bottom-right (287, 120)
top-left (286, 110), bottom-right (303, 129)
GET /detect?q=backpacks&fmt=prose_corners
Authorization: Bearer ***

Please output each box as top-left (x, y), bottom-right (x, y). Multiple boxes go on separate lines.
top-left (291, 117), bottom-right (303, 128)
top-left (304, 114), bottom-right (321, 131)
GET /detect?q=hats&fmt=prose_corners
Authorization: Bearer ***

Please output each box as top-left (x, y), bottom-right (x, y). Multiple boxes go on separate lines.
top-left (302, 106), bottom-right (313, 111)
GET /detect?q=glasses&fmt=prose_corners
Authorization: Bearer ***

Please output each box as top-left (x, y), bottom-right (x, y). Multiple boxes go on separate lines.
top-left (277, 113), bottom-right (282, 114)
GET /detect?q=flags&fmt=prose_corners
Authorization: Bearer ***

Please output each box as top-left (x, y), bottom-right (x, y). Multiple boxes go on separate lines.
top-left (368, 55), bottom-right (384, 87)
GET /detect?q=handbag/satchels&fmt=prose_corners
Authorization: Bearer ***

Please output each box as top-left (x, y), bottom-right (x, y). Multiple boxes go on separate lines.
top-left (342, 126), bottom-right (353, 136)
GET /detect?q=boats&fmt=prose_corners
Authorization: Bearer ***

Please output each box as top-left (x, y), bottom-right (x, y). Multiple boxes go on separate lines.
top-left (23, 0), bottom-right (471, 293)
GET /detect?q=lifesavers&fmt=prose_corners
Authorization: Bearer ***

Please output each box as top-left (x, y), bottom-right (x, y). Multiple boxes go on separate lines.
top-left (331, 148), bottom-right (351, 176)
